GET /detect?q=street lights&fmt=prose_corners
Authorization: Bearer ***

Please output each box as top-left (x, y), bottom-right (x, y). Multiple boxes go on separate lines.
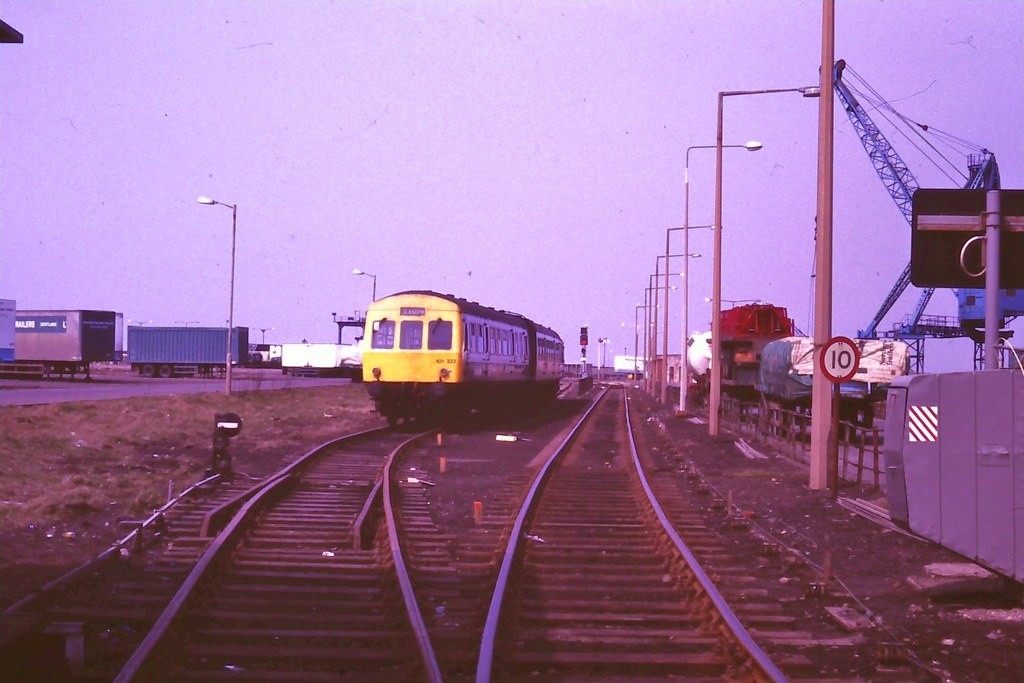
top-left (647, 272), bottom-right (684, 393)
top-left (353, 270), bottom-right (377, 302)
top-left (642, 286), bottom-right (679, 391)
top-left (651, 252), bottom-right (704, 398)
top-left (708, 85), bottom-right (822, 437)
top-left (634, 304), bottom-right (661, 384)
top-left (679, 142), bottom-right (763, 414)
top-left (657, 224), bottom-right (723, 404)
top-left (196, 196), bottom-right (238, 392)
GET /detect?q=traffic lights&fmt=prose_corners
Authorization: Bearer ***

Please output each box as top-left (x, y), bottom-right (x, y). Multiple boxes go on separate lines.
top-left (579, 335), bottom-right (588, 346)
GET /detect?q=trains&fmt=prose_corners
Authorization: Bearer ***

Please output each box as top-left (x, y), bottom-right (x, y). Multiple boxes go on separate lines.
top-left (360, 290), bottom-right (565, 427)
